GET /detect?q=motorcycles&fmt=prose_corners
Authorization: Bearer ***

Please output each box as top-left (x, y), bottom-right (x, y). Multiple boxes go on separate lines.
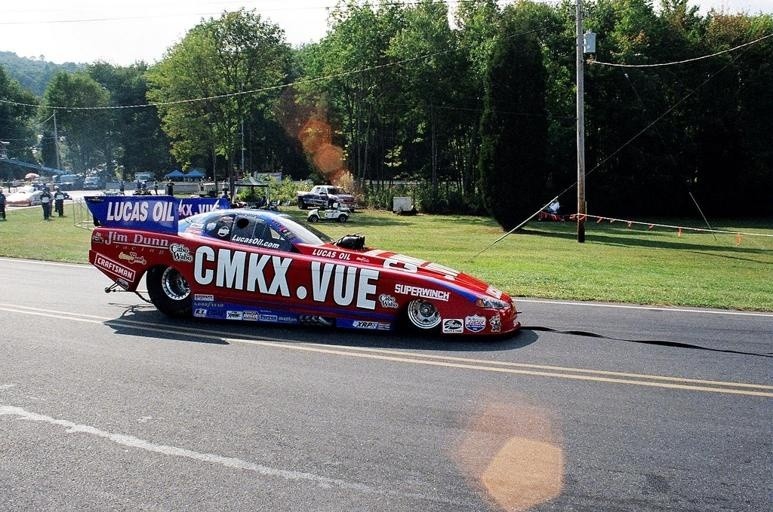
top-left (305, 209), bottom-right (350, 223)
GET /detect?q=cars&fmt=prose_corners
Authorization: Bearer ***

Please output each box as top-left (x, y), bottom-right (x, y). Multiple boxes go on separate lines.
top-left (296, 184), bottom-right (356, 211)
top-left (6, 175), bottom-right (105, 207)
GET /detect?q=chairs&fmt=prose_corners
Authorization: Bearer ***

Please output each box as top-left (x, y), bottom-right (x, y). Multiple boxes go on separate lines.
top-left (255, 224), bottom-right (286, 250)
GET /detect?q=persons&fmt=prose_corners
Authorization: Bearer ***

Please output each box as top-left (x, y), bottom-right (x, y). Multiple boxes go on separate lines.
top-left (45, 185), bottom-right (53, 215)
top-left (40, 188), bottom-right (51, 219)
top-left (135, 179), bottom-right (142, 193)
top-left (0, 189), bottom-right (7, 220)
top-left (118, 180), bottom-right (125, 193)
top-left (207, 176), bottom-right (214, 182)
top-left (200, 175), bottom-right (207, 183)
top-left (53, 187), bottom-right (65, 217)
top-left (209, 188), bottom-right (218, 197)
top-left (221, 191), bottom-right (230, 201)
top-left (167, 179), bottom-right (175, 196)
top-left (221, 179), bottom-right (229, 191)
top-left (198, 180), bottom-right (204, 191)
top-left (153, 178), bottom-right (159, 195)
top-left (541, 197), bottom-right (560, 214)
top-left (141, 180), bottom-right (147, 189)
top-left (254, 195), bottom-right (267, 209)
